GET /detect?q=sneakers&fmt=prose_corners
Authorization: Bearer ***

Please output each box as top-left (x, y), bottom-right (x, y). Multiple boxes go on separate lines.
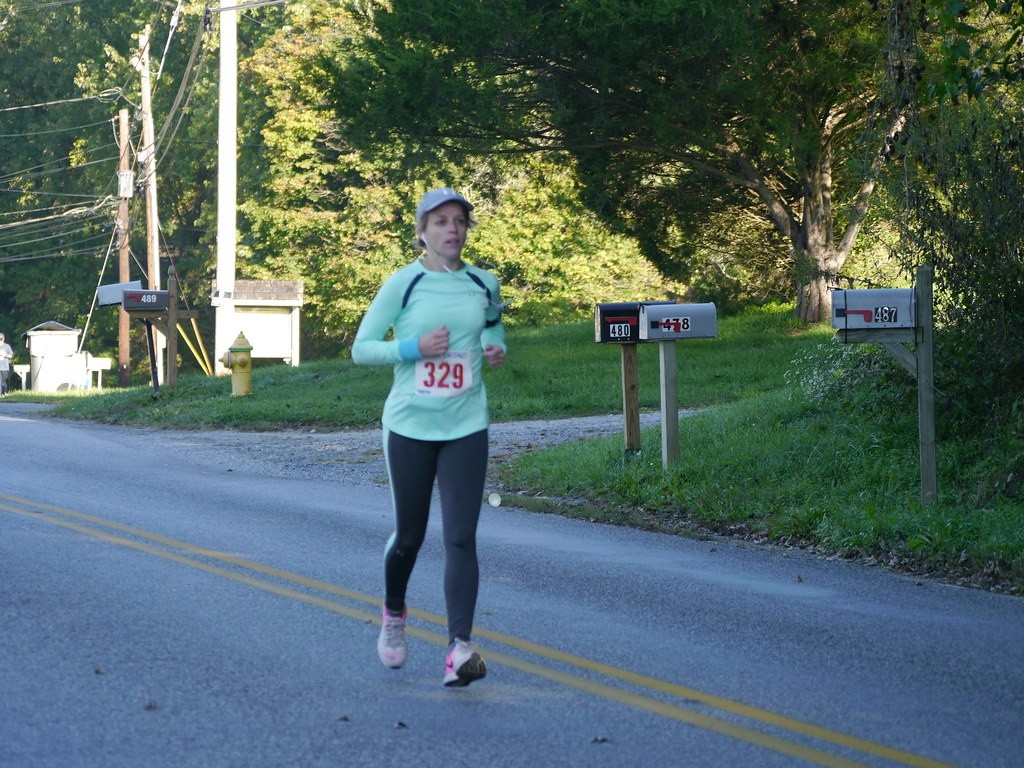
top-left (443, 637), bottom-right (486, 688)
top-left (378, 603), bottom-right (407, 667)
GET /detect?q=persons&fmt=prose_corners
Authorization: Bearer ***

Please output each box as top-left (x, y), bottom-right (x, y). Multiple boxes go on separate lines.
top-left (0, 333), bottom-right (14, 395)
top-left (351, 188), bottom-right (508, 688)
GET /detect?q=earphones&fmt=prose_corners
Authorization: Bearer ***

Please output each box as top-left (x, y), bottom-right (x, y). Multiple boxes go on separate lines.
top-left (421, 232), bottom-right (427, 243)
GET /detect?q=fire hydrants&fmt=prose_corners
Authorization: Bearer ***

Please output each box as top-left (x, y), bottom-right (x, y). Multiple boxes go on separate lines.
top-left (219, 331), bottom-right (253, 397)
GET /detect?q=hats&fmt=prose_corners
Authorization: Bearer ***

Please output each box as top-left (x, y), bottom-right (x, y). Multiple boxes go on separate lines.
top-left (416, 188), bottom-right (473, 223)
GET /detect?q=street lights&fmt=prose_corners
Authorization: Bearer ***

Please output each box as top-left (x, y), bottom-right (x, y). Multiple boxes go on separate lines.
top-left (64, 194), bottom-right (130, 388)
